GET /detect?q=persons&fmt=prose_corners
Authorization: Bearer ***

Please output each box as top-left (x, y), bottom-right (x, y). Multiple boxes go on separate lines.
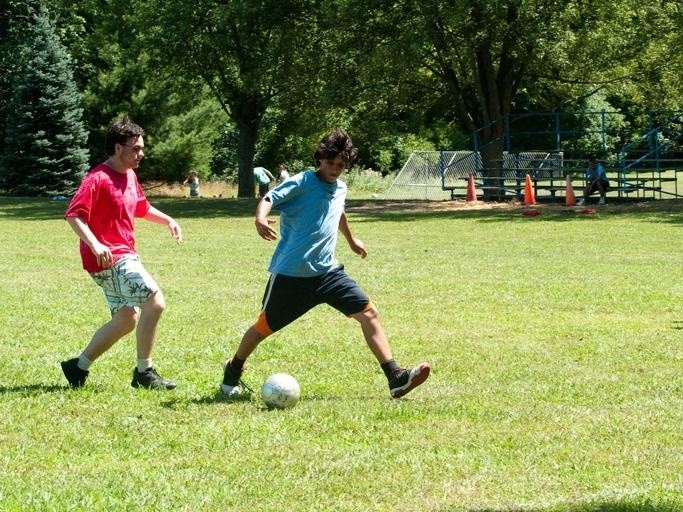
top-left (60, 120), bottom-right (184, 390)
top-left (221, 130), bottom-right (431, 398)
top-left (253, 166), bottom-right (277, 196)
top-left (576, 156), bottom-right (609, 206)
top-left (279, 162), bottom-right (289, 183)
top-left (183, 172), bottom-right (200, 196)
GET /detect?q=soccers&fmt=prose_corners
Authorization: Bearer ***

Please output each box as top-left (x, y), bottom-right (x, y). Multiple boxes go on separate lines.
top-left (260, 374), bottom-right (300, 410)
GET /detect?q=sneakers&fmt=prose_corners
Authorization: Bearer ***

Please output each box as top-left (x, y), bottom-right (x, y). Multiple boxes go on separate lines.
top-left (131, 367), bottom-right (177, 390)
top-left (222, 358), bottom-right (241, 395)
top-left (598, 197), bottom-right (605, 204)
top-left (576, 198), bottom-right (586, 206)
top-left (388, 362), bottom-right (430, 398)
top-left (61, 358), bottom-right (89, 387)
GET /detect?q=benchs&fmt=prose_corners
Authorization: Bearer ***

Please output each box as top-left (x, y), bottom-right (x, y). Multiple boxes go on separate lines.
top-left (443, 148), bottom-right (682, 203)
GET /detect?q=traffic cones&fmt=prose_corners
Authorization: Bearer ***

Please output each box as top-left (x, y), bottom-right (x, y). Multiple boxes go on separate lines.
top-left (520, 173), bottom-right (537, 204)
top-left (563, 172), bottom-right (577, 205)
top-left (465, 170), bottom-right (477, 202)
